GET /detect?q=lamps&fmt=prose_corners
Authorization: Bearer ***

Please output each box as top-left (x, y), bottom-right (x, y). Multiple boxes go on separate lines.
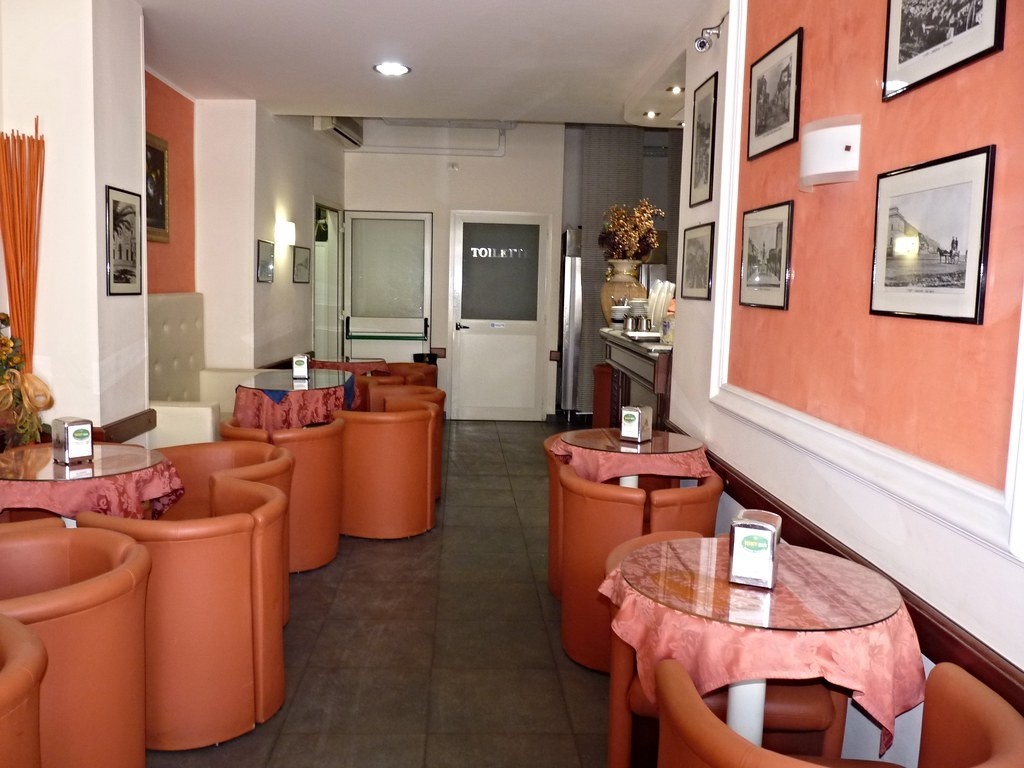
top-left (798, 113), bottom-right (863, 193)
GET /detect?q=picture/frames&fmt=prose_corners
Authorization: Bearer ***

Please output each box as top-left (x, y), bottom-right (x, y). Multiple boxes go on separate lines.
top-left (738, 196), bottom-right (796, 309)
top-left (257, 238), bottom-right (275, 283)
top-left (293, 246), bottom-right (311, 284)
top-left (880, 0), bottom-right (1007, 104)
top-left (869, 141), bottom-right (999, 329)
top-left (747, 27), bottom-right (805, 160)
top-left (147, 131), bottom-right (169, 243)
top-left (105, 184), bottom-right (143, 297)
top-left (688, 71), bottom-right (719, 208)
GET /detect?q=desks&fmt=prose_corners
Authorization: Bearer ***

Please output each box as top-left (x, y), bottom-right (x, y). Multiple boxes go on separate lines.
top-left (598, 327), bottom-right (672, 430)
top-left (307, 356), bottom-right (391, 376)
top-left (231, 369), bottom-right (362, 428)
top-left (551, 427), bottom-right (716, 488)
top-left (0, 443), bottom-right (186, 528)
top-left (596, 535), bottom-right (906, 751)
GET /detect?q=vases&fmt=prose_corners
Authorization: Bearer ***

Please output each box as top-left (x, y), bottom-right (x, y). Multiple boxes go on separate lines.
top-left (600, 259), bottom-right (648, 326)
top-left (680, 221), bottom-right (715, 301)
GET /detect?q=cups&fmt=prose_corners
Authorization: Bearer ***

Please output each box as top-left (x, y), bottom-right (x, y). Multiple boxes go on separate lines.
top-left (637, 316), bottom-right (652, 332)
top-left (610, 305), bottom-right (632, 331)
top-left (623, 316), bottom-right (637, 332)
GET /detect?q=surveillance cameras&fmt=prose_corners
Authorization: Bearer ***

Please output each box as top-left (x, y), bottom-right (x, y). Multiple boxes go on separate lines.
top-left (695, 37), bottom-right (713, 53)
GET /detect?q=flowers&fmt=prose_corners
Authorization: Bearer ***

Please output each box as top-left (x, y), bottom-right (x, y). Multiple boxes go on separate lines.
top-left (598, 196), bottom-right (665, 260)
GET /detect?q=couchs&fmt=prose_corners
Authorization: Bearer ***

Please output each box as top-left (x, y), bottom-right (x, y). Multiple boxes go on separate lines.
top-left (542, 433), bottom-right (1024, 768)
top-left (0, 363), bottom-right (447, 768)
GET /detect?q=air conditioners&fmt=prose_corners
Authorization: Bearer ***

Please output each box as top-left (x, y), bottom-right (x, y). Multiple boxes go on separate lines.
top-left (313, 116), bottom-right (364, 150)
